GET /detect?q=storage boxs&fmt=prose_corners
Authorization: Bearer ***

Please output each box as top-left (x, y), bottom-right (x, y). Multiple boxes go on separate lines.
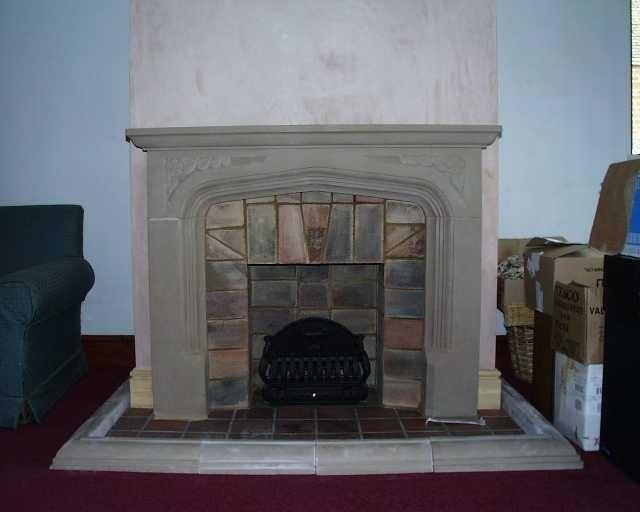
top-left (499, 157), bottom-right (640, 485)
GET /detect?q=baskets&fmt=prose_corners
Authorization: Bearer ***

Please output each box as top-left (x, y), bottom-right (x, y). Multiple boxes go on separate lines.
top-left (507, 325), bottom-right (537, 384)
top-left (504, 303), bottom-right (537, 327)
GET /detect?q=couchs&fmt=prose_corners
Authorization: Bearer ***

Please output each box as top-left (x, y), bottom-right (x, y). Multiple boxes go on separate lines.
top-left (0, 204), bottom-right (95, 431)
